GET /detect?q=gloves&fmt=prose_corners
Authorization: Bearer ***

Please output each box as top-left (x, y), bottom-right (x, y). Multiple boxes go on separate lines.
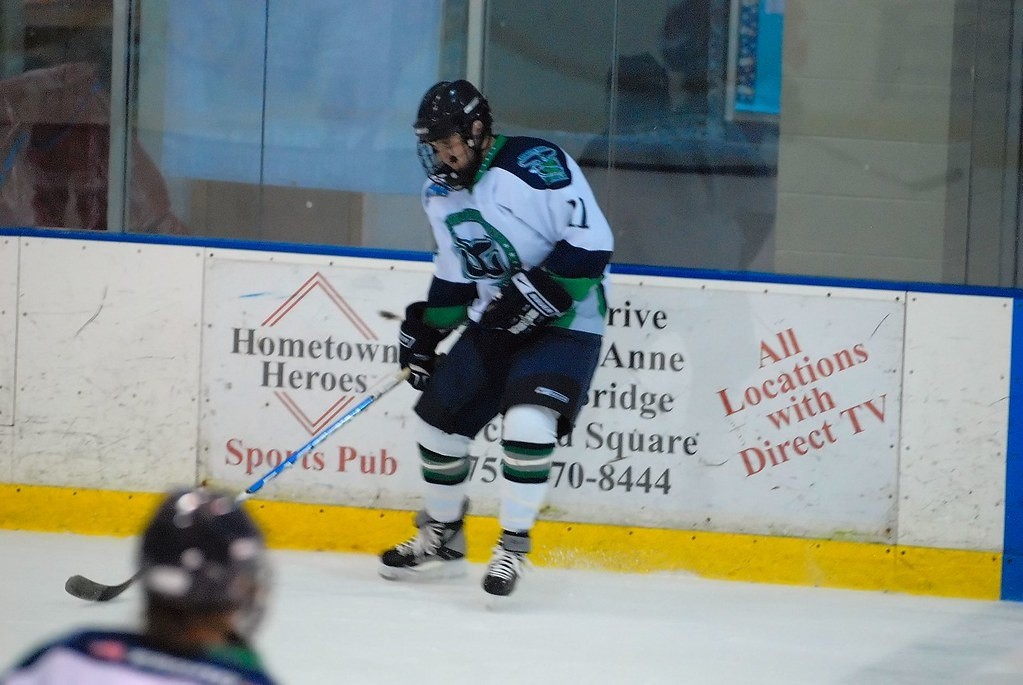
top-left (399, 302), bottom-right (449, 390)
top-left (475, 266), bottom-right (572, 360)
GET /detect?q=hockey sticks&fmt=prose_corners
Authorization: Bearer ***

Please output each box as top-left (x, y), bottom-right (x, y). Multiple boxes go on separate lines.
top-left (65, 318), bottom-right (474, 602)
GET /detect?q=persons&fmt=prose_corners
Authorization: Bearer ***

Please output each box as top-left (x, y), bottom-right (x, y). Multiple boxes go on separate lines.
top-left (380, 79), bottom-right (614, 598)
top-left (0, 487), bottom-right (279, 685)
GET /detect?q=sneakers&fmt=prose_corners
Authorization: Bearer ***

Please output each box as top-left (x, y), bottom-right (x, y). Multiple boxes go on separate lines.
top-left (482, 529), bottom-right (531, 611)
top-left (378, 495), bottom-right (470, 583)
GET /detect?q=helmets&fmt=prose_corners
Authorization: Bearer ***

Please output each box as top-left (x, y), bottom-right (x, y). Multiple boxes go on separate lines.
top-left (413, 79), bottom-right (492, 192)
top-left (141, 489), bottom-right (266, 638)
